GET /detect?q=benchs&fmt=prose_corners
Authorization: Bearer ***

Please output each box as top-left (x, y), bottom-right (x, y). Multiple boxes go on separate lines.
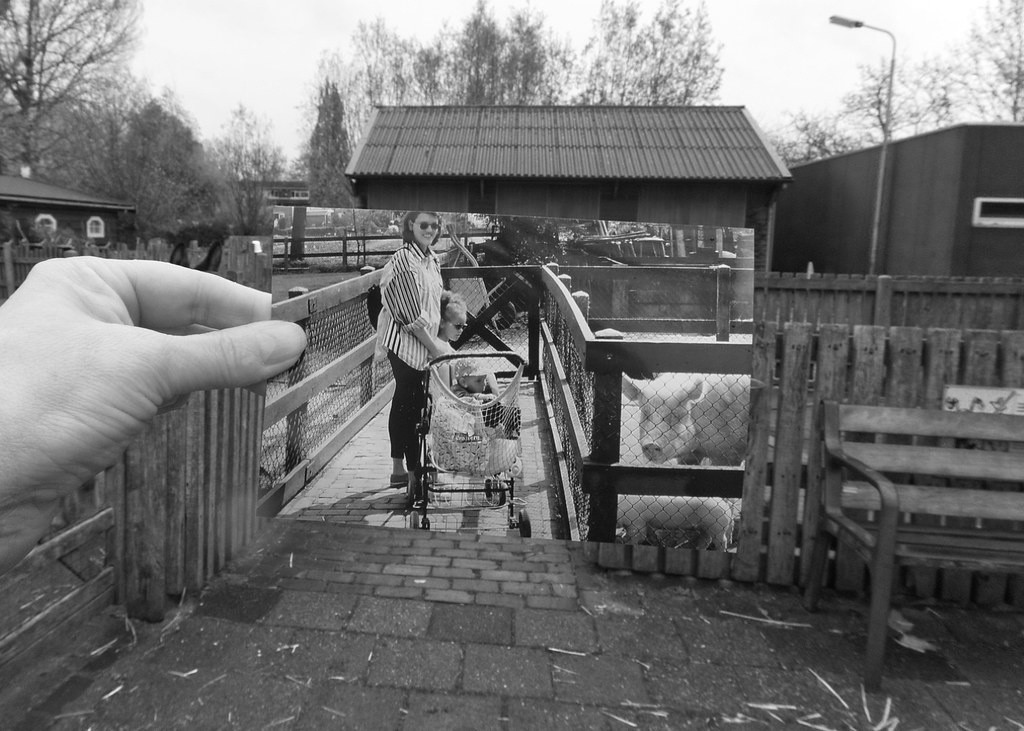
top-left (804, 396), bottom-right (1024, 665)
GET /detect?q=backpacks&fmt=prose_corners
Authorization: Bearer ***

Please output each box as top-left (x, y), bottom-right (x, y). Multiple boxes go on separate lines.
top-left (366, 284), bottom-right (383, 331)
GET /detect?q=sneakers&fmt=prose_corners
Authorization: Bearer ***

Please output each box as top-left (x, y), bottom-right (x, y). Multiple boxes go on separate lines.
top-left (389, 473), bottom-right (409, 487)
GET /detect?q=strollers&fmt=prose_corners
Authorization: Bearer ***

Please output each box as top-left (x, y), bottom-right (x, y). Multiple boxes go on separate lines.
top-left (407, 350), bottom-right (531, 539)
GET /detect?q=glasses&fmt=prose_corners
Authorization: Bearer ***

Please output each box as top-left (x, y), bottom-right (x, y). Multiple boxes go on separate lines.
top-left (443, 315), bottom-right (467, 330)
top-left (413, 221), bottom-right (441, 231)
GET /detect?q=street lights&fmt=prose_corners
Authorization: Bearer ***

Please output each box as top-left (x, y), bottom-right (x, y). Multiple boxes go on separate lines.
top-left (829, 15), bottom-right (897, 275)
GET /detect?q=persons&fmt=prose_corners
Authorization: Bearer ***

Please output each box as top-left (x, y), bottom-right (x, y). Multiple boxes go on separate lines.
top-left (446, 355), bottom-right (520, 440)
top-left (377, 210), bottom-right (448, 504)
top-left (429, 289), bottom-right (468, 392)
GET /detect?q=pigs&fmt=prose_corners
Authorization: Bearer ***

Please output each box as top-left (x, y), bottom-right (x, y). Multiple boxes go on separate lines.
top-left (621, 370), bottom-right (751, 466)
top-left (618, 494), bottom-right (732, 550)
